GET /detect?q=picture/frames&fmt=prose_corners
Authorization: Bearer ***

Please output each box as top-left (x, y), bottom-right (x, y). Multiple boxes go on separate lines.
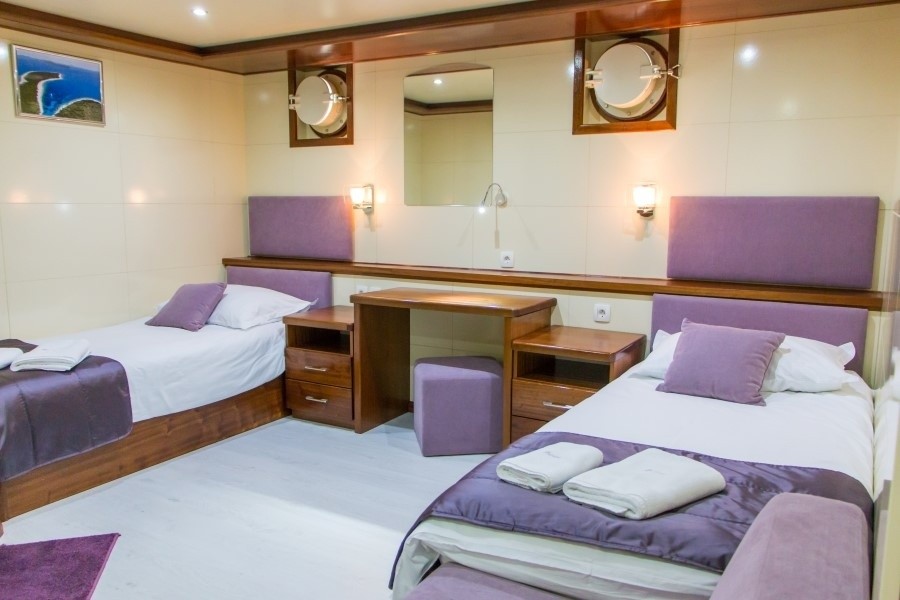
top-left (8, 42), bottom-right (106, 126)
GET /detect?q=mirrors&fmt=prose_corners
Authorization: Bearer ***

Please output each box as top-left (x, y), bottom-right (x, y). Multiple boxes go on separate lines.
top-left (402, 62), bottom-right (495, 207)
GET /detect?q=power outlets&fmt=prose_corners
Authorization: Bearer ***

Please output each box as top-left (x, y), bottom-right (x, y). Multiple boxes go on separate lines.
top-left (593, 304), bottom-right (610, 323)
top-left (357, 286), bottom-right (367, 293)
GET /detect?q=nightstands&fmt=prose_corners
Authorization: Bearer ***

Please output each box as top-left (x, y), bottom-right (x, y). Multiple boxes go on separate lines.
top-left (511, 323), bottom-right (647, 447)
top-left (283, 305), bottom-right (355, 430)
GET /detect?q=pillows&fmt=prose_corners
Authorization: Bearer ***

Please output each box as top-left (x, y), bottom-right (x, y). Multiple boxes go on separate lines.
top-left (633, 334), bottom-right (855, 391)
top-left (153, 283), bottom-right (319, 330)
top-left (145, 280), bottom-right (225, 329)
top-left (657, 318), bottom-right (786, 405)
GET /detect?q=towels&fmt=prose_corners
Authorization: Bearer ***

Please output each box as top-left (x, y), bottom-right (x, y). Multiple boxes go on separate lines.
top-left (0, 347), bottom-right (24, 370)
top-left (563, 448), bottom-right (726, 521)
top-left (10, 338), bottom-right (93, 373)
top-left (496, 441), bottom-right (604, 494)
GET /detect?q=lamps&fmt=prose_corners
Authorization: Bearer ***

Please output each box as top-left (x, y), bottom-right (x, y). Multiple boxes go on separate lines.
top-left (478, 183), bottom-right (507, 215)
top-left (350, 183), bottom-right (374, 213)
top-left (632, 182), bottom-right (657, 218)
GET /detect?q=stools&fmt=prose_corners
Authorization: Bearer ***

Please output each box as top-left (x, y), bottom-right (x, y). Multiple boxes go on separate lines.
top-left (412, 355), bottom-right (505, 458)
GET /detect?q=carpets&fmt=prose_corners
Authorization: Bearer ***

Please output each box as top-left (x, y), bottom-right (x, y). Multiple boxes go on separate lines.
top-left (0, 532), bottom-right (120, 599)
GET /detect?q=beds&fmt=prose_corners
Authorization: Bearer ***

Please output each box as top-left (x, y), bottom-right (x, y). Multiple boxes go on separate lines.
top-left (383, 293), bottom-right (900, 600)
top-left (1, 272), bottom-right (336, 525)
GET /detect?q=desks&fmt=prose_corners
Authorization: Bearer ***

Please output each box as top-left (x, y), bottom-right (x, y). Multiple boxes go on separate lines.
top-left (351, 287), bottom-right (556, 452)
